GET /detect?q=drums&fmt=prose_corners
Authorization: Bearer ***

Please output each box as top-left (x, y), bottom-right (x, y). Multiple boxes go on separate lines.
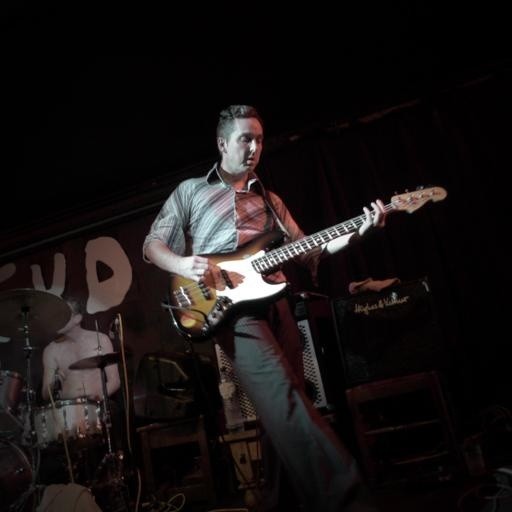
top-left (1, 439), bottom-right (33, 508)
top-left (1, 370), bottom-right (24, 416)
top-left (34, 398), bottom-right (111, 450)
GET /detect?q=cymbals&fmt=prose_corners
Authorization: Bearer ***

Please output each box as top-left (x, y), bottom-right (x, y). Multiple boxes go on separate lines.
top-left (68, 353), bottom-right (133, 369)
top-left (0, 289), bottom-right (70, 338)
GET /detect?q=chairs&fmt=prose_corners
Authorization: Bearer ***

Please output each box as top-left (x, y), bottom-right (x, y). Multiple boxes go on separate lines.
top-left (328, 276), bottom-right (470, 499)
top-left (134, 350), bottom-right (225, 510)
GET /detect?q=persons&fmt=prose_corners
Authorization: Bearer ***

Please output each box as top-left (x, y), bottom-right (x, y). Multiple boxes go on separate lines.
top-left (139, 103), bottom-right (387, 510)
top-left (38, 298), bottom-right (122, 403)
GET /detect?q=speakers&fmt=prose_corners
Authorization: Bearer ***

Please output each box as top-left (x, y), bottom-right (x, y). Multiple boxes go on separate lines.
top-left (331, 276), bottom-right (448, 388)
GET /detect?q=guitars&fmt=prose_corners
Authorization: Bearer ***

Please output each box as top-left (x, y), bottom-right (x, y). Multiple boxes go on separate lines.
top-left (166, 187), bottom-right (449, 343)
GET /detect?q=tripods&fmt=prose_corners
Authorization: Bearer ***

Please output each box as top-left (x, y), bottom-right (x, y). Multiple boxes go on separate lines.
top-left (86, 347), bottom-right (149, 502)
